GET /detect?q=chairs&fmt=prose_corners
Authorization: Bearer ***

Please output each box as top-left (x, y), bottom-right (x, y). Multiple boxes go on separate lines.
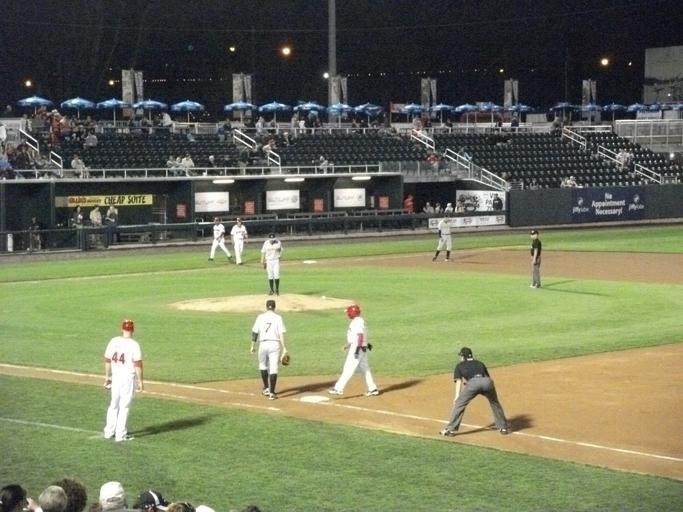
top-left (28, 131), bottom-right (683, 190)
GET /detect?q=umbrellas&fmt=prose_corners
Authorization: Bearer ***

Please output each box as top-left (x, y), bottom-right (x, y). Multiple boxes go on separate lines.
top-left (17, 96), bottom-right (683, 126)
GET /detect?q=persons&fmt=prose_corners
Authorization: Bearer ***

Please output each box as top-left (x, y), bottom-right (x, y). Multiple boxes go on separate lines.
top-left (208, 217), bottom-right (233, 260)
top-left (327, 305), bottom-right (380, 397)
top-left (439, 347), bottom-right (509, 437)
top-left (260, 233), bottom-right (283, 294)
top-left (252, 299), bottom-right (288, 400)
top-left (1, 478), bottom-right (261, 510)
top-left (102, 319), bottom-right (144, 441)
top-left (530, 231), bottom-right (542, 288)
top-left (432, 216), bottom-right (453, 262)
top-left (1, 119), bottom-right (637, 249)
top-left (230, 218), bottom-right (248, 265)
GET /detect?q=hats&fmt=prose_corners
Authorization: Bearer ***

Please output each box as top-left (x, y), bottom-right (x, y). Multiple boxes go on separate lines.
top-left (458, 347), bottom-right (472, 358)
top-left (99, 480), bottom-right (123, 509)
top-left (133, 490), bottom-right (164, 509)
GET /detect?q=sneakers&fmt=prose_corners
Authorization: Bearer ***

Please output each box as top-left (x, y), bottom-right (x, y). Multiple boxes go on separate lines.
top-left (365, 390), bottom-right (379, 396)
top-left (328, 389), bottom-right (344, 395)
top-left (263, 388), bottom-right (278, 400)
top-left (439, 428), bottom-right (454, 437)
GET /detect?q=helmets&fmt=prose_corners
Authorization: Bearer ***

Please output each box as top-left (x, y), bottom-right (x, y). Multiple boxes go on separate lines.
top-left (346, 306), bottom-right (360, 318)
top-left (122, 320), bottom-right (133, 331)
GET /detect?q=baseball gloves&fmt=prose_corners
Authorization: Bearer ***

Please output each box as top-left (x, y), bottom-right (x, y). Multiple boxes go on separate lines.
top-left (279, 353), bottom-right (291, 367)
